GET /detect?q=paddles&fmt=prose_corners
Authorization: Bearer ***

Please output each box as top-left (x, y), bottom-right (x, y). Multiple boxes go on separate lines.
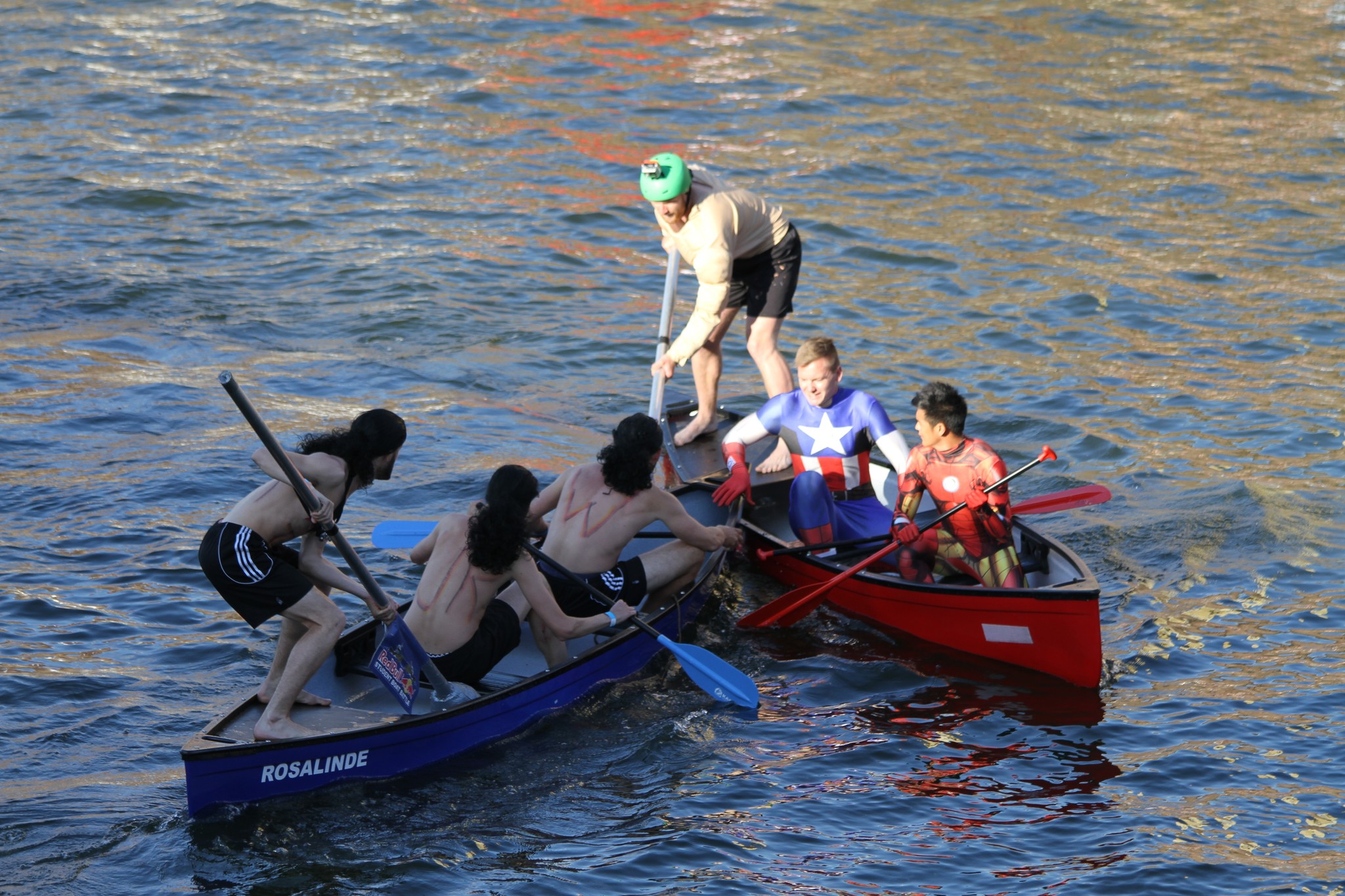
top-left (475, 501), bottom-right (758, 710)
top-left (735, 445), bottom-right (1112, 628)
top-left (370, 519), bottom-right (745, 552)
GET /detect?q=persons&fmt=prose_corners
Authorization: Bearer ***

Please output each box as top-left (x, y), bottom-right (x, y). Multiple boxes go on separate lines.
top-left (639, 153), bottom-right (803, 474)
top-left (891, 380), bottom-right (1029, 588)
top-left (403, 464), bottom-right (637, 682)
top-left (523, 413), bottom-right (744, 616)
top-left (197, 409), bottom-right (406, 739)
top-left (712, 338), bottom-right (935, 586)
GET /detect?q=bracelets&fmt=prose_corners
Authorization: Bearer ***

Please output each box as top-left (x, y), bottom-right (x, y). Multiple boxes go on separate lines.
top-left (605, 611), bottom-right (617, 627)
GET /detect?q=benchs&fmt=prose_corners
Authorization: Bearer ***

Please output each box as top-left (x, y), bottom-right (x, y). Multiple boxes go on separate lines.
top-left (358, 653), bottom-right (524, 701)
top-left (802, 509), bottom-right (940, 559)
top-left (508, 579), bottom-right (650, 639)
top-left (704, 460), bottom-right (802, 492)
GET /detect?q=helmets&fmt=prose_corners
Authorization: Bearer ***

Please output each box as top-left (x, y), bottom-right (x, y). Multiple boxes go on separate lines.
top-left (639, 153), bottom-right (692, 201)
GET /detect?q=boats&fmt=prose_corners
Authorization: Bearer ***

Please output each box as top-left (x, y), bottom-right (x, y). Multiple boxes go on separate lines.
top-left (660, 395), bottom-right (1103, 690)
top-left (177, 460), bottom-right (754, 811)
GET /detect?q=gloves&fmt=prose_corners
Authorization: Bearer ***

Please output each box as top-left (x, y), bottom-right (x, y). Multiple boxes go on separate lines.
top-left (897, 523), bottom-right (923, 545)
top-left (712, 453), bottom-right (756, 507)
top-left (965, 485), bottom-right (988, 510)
